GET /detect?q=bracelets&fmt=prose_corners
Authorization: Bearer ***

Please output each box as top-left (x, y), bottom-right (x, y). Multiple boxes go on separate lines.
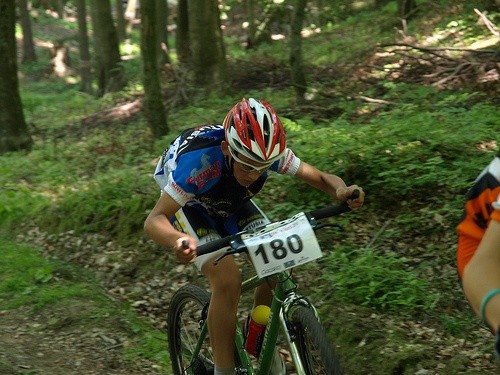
top-left (477, 289), bottom-right (500, 328)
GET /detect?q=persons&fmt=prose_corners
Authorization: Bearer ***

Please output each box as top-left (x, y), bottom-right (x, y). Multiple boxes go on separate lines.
top-left (143, 97), bottom-right (366, 375)
top-left (453, 151), bottom-right (500, 341)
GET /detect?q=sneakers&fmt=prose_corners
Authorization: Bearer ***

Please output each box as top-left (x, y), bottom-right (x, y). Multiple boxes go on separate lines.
top-left (270, 347), bottom-right (287, 375)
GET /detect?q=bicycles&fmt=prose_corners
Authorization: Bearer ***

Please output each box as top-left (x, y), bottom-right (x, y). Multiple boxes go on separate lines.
top-left (167, 189), bottom-right (360, 375)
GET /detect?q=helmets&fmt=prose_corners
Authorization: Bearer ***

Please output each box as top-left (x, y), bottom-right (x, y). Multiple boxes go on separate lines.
top-left (223, 97), bottom-right (286, 164)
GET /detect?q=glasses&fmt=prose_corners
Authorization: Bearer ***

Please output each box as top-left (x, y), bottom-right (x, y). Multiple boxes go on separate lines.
top-left (227, 146), bottom-right (274, 173)
